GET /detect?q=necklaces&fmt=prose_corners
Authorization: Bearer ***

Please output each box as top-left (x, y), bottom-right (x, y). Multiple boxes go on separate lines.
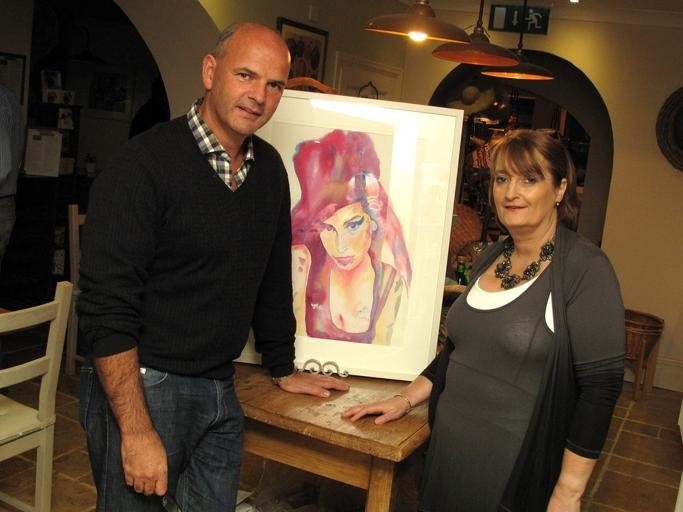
top-left (495, 237), bottom-right (554, 289)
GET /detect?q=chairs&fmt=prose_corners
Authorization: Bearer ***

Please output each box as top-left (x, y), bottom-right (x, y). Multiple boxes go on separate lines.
top-left (0, 203), bottom-right (89, 512)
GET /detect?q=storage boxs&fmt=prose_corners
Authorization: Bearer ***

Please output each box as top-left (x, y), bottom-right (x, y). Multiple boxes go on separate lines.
top-left (23, 125), bottom-right (63, 179)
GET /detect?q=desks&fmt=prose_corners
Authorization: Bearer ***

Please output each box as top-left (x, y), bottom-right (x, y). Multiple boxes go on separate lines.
top-left (227, 361), bottom-right (433, 512)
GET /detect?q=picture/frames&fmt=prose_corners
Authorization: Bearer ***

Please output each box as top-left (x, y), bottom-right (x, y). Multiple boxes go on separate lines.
top-left (0, 51), bottom-right (26, 105)
top-left (276, 16), bottom-right (329, 85)
top-left (232, 87), bottom-right (462, 385)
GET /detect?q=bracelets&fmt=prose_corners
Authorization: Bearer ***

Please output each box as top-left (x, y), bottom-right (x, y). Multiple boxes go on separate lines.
top-left (272, 377), bottom-right (287, 385)
top-left (395, 394), bottom-right (413, 411)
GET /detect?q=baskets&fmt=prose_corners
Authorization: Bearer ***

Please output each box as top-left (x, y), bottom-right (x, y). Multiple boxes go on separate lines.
top-left (440, 306), bottom-right (449, 337)
top-left (624, 309), bottom-right (665, 362)
top-left (448, 203), bottom-right (493, 259)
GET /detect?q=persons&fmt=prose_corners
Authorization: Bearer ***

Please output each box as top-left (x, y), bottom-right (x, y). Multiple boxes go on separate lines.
top-left (290, 130), bottom-right (413, 347)
top-left (0, 81), bottom-right (27, 264)
top-left (75, 22), bottom-right (351, 512)
top-left (340, 126), bottom-right (625, 512)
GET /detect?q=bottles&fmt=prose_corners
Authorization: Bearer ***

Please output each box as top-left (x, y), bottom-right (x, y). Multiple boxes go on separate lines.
top-left (455, 255), bottom-right (468, 286)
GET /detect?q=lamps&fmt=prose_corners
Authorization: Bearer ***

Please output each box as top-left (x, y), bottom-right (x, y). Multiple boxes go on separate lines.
top-left (363, 1), bottom-right (555, 81)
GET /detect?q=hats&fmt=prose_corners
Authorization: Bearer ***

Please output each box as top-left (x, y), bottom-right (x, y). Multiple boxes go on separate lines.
top-left (444, 78), bottom-right (496, 115)
top-left (656, 86), bottom-right (683, 172)
